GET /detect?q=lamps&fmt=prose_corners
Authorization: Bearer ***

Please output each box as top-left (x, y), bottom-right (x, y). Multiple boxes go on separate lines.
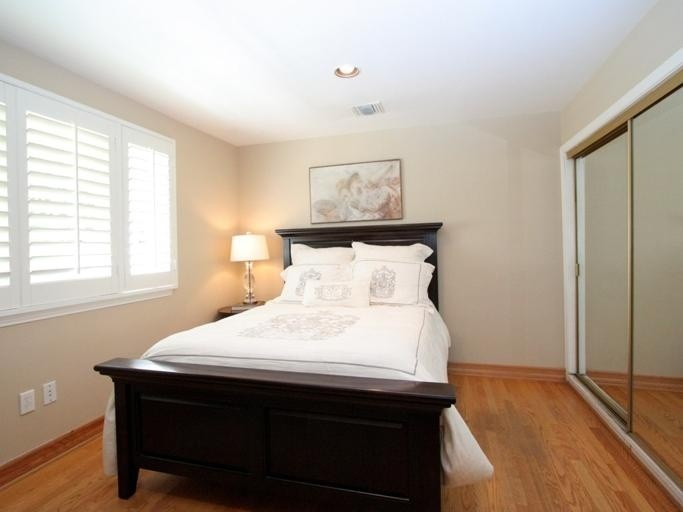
top-left (230, 231), bottom-right (270, 305)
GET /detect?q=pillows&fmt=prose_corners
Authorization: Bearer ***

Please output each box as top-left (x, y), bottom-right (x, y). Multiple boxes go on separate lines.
top-left (272, 241), bottom-right (436, 309)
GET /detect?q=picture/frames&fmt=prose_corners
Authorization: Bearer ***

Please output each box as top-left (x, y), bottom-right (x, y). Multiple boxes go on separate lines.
top-left (309, 158), bottom-right (403, 224)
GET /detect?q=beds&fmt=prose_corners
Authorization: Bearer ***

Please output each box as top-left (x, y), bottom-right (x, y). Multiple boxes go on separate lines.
top-left (93, 222), bottom-right (457, 512)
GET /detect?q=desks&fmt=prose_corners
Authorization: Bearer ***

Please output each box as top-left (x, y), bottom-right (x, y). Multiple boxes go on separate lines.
top-left (218, 301), bottom-right (265, 319)
